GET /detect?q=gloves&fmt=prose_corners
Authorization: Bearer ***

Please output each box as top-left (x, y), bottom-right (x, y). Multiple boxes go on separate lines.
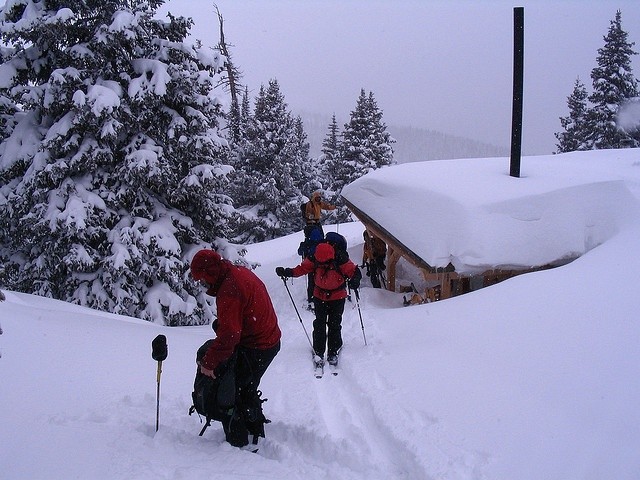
top-left (348, 264), bottom-right (362, 289)
top-left (275, 266), bottom-right (292, 277)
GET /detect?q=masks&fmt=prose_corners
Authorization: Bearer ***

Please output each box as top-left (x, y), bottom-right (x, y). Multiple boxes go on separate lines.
top-left (200, 282), bottom-right (211, 288)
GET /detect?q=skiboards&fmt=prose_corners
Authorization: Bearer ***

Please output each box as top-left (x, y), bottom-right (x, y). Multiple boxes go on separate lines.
top-left (315, 365), bottom-right (338, 379)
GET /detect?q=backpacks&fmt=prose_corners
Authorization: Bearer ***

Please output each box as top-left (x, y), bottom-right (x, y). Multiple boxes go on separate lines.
top-left (189, 339), bottom-right (271, 437)
top-left (300, 201), bottom-right (314, 219)
top-left (307, 232), bottom-right (352, 304)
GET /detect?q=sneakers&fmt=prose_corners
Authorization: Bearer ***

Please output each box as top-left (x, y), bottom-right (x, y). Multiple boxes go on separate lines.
top-left (327, 350), bottom-right (338, 364)
top-left (314, 354), bottom-right (325, 367)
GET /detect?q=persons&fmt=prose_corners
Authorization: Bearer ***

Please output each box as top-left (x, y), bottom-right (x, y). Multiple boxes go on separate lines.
top-left (191, 250), bottom-right (281, 449)
top-left (276, 242), bottom-right (362, 380)
top-left (362, 230), bottom-right (387, 287)
top-left (305, 191), bottom-right (337, 229)
top-left (298, 229), bottom-right (328, 310)
